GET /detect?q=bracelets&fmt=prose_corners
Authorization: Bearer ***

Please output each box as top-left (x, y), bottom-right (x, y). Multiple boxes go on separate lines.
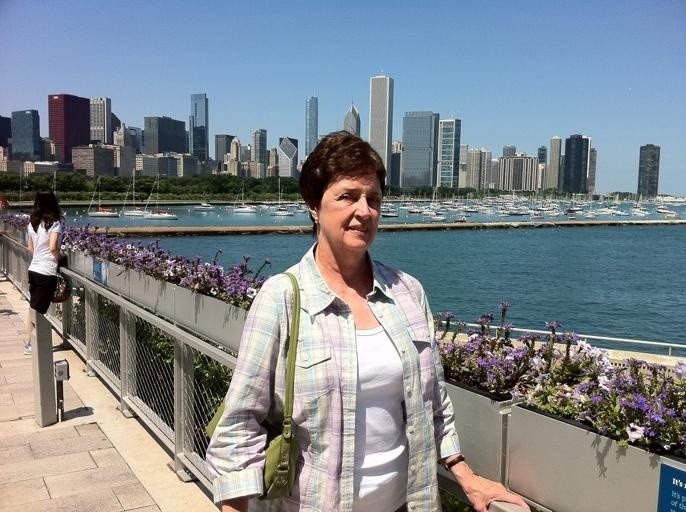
top-left (444, 454), bottom-right (465, 471)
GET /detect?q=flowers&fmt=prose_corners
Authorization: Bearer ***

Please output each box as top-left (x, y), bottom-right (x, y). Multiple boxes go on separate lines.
top-left (1, 211), bottom-right (685, 463)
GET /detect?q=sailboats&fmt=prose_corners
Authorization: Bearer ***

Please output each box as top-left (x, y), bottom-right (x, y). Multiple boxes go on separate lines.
top-left (13, 165), bottom-right (177, 220)
top-left (224, 176), bottom-right (309, 216)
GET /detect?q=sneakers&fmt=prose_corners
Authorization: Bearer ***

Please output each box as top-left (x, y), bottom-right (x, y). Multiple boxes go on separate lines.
top-left (23, 344), bottom-right (33, 356)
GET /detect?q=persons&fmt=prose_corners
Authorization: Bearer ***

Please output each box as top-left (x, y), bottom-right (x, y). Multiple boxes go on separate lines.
top-left (204, 130), bottom-right (534, 512)
top-left (24, 186), bottom-right (66, 351)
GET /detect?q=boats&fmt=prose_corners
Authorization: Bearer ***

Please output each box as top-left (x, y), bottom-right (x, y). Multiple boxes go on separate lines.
top-left (194, 203), bottom-right (216, 211)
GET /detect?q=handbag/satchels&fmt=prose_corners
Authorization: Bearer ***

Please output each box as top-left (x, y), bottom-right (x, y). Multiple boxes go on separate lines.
top-left (205, 270), bottom-right (306, 502)
top-left (51, 271), bottom-right (70, 304)
